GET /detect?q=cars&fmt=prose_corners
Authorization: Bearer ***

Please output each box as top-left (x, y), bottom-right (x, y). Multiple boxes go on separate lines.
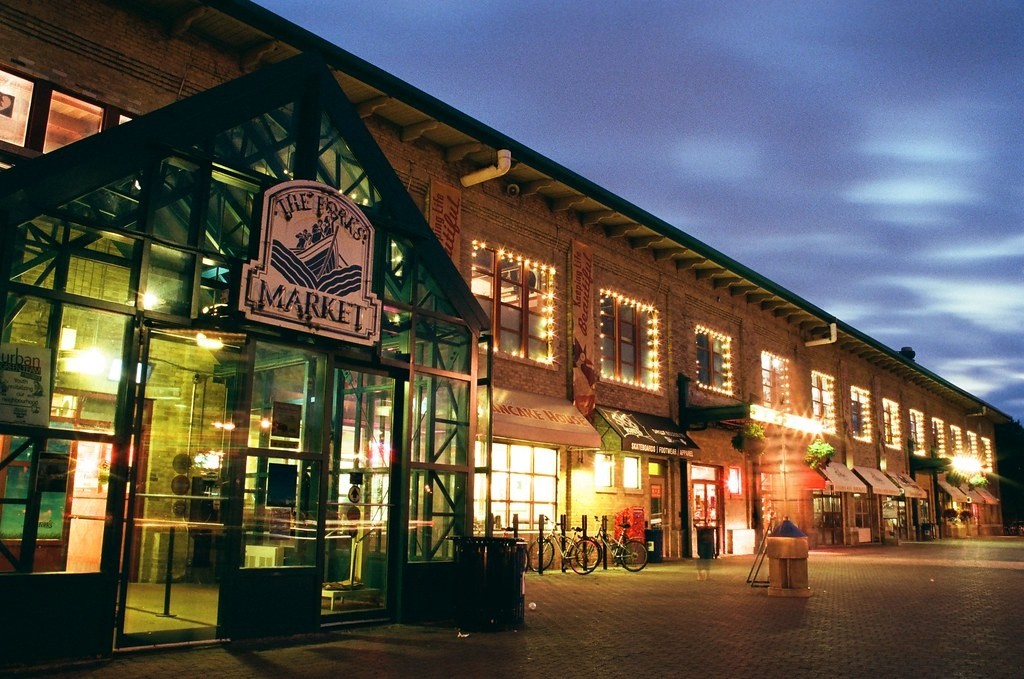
top-left (1010, 521), bottom-right (1024, 536)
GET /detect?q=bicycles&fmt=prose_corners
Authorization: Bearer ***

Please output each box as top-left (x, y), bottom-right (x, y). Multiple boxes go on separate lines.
top-left (476, 527), bottom-right (528, 573)
top-left (527, 516), bottom-right (600, 575)
top-left (575, 516), bottom-right (648, 572)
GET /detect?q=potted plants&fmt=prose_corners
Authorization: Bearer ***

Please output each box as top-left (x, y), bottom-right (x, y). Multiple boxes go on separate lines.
top-left (804, 438), bottom-right (836, 470)
top-left (967, 474), bottom-right (990, 491)
top-left (946, 471), bottom-right (968, 488)
top-left (731, 423), bottom-right (766, 457)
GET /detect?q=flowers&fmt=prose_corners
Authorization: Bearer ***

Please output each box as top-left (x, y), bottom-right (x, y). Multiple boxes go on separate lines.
top-left (942, 509), bottom-right (958, 518)
top-left (958, 510), bottom-right (972, 520)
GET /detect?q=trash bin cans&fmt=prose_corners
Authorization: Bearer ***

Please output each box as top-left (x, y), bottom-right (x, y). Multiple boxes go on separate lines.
top-left (696, 526), bottom-right (715, 559)
top-left (446, 535), bottom-right (528, 633)
top-left (921, 523), bottom-right (933, 541)
top-left (644, 528), bottom-right (663, 563)
